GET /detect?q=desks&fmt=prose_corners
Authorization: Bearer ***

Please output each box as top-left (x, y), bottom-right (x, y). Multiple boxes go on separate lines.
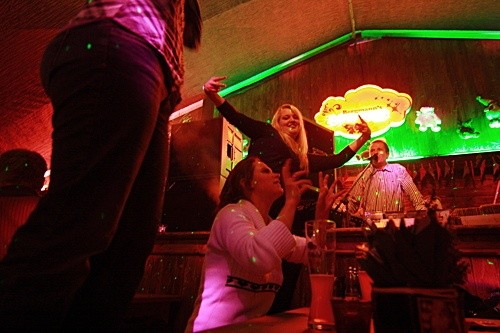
top-left (194, 307), bottom-right (500, 332)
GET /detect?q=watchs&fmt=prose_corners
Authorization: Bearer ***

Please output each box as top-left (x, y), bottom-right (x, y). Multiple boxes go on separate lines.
top-left (202, 84), bottom-right (212, 96)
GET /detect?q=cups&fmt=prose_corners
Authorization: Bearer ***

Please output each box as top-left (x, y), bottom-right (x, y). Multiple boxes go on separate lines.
top-left (303, 220), bottom-right (338, 329)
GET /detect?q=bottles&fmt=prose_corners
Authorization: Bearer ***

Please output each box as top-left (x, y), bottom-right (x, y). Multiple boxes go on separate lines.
top-left (346, 265), bottom-right (359, 294)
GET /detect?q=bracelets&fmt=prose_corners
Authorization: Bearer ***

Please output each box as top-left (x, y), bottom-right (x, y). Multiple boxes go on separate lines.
top-left (275, 214), bottom-right (292, 232)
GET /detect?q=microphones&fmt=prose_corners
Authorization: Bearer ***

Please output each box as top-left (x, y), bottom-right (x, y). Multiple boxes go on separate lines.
top-left (368, 155), bottom-right (378, 160)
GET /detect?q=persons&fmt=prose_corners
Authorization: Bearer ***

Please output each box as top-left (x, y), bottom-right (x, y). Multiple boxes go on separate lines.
top-left (0, 149), bottom-right (47, 260)
top-left (333, 140), bottom-right (427, 214)
top-left (203, 76), bottom-right (371, 315)
top-left (0, 0), bottom-right (201, 333)
top-left (185, 157), bottom-right (347, 333)
